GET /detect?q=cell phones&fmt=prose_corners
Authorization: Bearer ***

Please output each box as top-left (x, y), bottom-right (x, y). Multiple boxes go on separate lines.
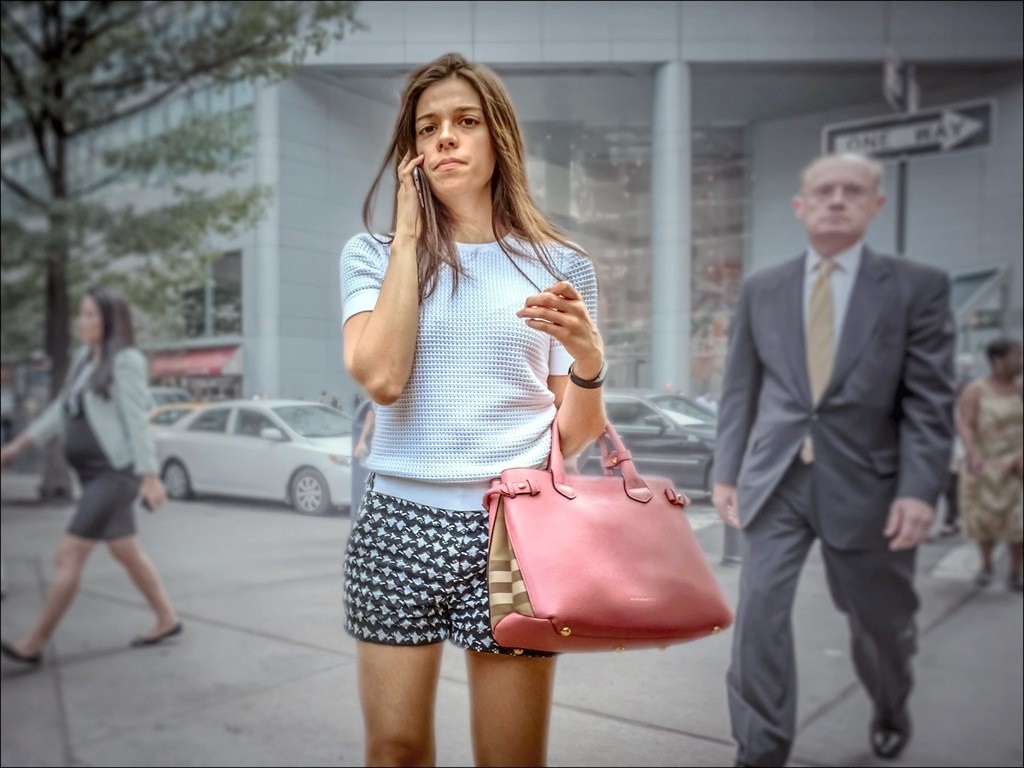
top-left (413, 166), bottom-right (425, 209)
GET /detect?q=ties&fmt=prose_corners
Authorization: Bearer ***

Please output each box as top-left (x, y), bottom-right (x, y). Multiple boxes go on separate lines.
top-left (801, 260), bottom-right (835, 464)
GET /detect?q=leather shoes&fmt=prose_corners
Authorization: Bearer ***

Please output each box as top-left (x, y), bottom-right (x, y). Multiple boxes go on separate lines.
top-left (870, 713), bottom-right (912, 759)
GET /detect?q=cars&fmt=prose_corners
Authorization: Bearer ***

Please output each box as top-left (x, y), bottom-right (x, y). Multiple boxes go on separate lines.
top-left (154, 400), bottom-right (356, 516)
top-left (145, 384), bottom-right (214, 432)
top-left (579, 388), bottom-right (718, 497)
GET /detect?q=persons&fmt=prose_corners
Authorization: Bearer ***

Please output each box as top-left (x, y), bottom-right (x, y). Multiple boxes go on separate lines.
top-left (1, 284), bottom-right (184, 665)
top-left (340, 53), bottom-right (608, 767)
top-left (711, 153), bottom-right (955, 767)
top-left (958, 339), bottom-right (1023, 592)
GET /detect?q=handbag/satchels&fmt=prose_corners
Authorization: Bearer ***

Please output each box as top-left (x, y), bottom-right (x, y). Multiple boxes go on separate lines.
top-left (482, 414), bottom-right (734, 654)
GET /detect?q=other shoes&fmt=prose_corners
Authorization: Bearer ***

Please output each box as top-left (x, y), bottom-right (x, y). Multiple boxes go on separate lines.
top-left (974, 566), bottom-right (994, 586)
top-left (131, 622), bottom-right (183, 647)
top-left (0, 640), bottom-right (44, 664)
top-left (1009, 572), bottom-right (1023, 591)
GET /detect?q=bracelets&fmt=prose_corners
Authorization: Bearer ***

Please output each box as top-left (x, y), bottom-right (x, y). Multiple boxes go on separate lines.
top-left (568, 360), bottom-right (608, 388)
